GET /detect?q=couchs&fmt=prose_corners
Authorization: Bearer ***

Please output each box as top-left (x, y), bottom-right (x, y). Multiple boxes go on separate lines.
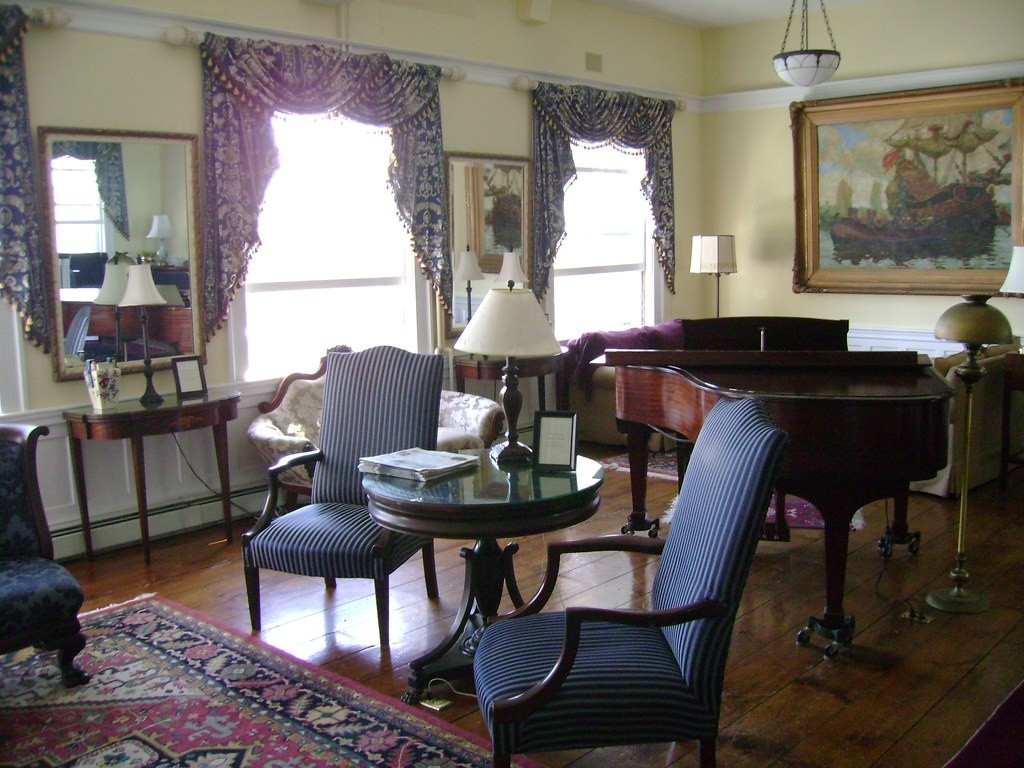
top-left (556, 319), bottom-right (684, 451)
top-left (910, 337), bottom-right (1024, 500)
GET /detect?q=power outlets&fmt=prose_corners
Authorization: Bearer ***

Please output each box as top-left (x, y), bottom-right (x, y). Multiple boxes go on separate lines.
top-left (419, 696), bottom-right (453, 712)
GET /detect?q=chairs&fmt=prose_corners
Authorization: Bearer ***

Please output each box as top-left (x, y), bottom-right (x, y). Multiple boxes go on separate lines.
top-left (238, 342), bottom-right (448, 655)
top-left (473, 396), bottom-right (794, 768)
top-left (0, 424), bottom-right (94, 693)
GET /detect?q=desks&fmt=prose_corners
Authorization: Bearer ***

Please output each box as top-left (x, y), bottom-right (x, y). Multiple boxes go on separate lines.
top-left (60, 388), bottom-right (243, 568)
top-left (455, 343), bottom-right (572, 409)
top-left (361, 446), bottom-right (605, 705)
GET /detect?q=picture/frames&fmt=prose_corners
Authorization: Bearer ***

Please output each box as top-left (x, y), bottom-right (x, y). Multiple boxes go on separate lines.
top-left (530, 410), bottom-right (578, 472)
top-left (172, 356), bottom-right (209, 399)
top-left (790, 76), bottom-right (1024, 298)
top-left (464, 163), bottom-right (524, 274)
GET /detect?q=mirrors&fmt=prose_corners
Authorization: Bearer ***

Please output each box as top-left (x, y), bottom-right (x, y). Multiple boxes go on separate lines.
top-left (441, 148), bottom-right (535, 341)
top-left (36, 123), bottom-right (208, 382)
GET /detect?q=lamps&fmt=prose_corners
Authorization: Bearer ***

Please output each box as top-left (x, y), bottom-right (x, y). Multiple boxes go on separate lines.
top-left (995, 245), bottom-right (1024, 354)
top-left (91, 251), bottom-right (135, 362)
top-left (924, 291), bottom-right (1013, 617)
top-left (455, 243), bottom-right (487, 323)
top-left (454, 281), bottom-right (564, 466)
top-left (145, 214), bottom-right (174, 261)
top-left (771, 0), bottom-right (842, 89)
top-left (494, 245), bottom-right (530, 290)
top-left (689, 235), bottom-right (737, 318)
top-left (117, 253), bottom-right (167, 406)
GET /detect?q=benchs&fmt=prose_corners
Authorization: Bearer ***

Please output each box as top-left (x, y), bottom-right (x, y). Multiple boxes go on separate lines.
top-left (242, 344), bottom-right (505, 546)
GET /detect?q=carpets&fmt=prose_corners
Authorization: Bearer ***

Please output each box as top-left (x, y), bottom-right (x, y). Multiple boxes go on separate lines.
top-left (658, 482), bottom-right (866, 534)
top-left (0, 592), bottom-right (550, 768)
top-left (601, 450), bottom-right (679, 481)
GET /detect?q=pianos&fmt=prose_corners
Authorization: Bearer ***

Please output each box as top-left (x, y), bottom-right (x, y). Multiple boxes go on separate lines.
top-left (586, 316), bottom-right (959, 660)
